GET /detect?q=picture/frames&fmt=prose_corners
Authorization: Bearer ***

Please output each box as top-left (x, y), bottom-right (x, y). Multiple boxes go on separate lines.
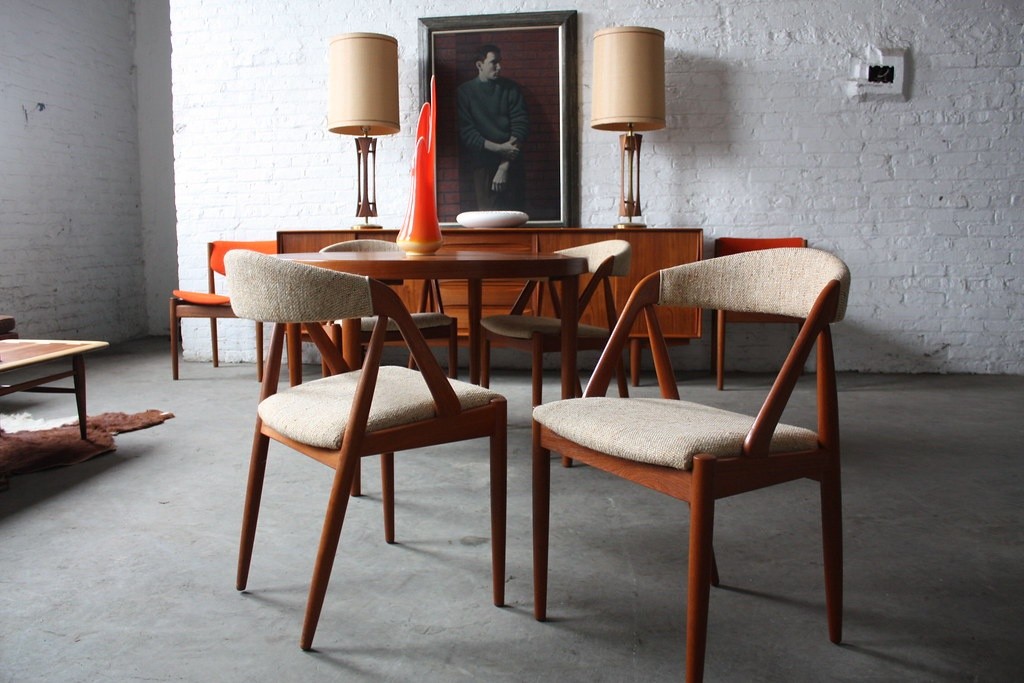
top-left (416, 10), bottom-right (579, 227)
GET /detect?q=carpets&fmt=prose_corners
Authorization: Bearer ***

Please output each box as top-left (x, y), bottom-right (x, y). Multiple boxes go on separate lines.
top-left (0, 409), bottom-right (176, 478)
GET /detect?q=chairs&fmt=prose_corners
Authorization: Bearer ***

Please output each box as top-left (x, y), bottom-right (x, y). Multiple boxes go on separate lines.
top-left (169, 240), bottom-right (277, 384)
top-left (223, 249), bottom-right (515, 650)
top-left (714, 235), bottom-right (824, 390)
top-left (533, 246), bottom-right (850, 682)
top-left (317, 237), bottom-right (458, 382)
top-left (479, 238), bottom-right (631, 399)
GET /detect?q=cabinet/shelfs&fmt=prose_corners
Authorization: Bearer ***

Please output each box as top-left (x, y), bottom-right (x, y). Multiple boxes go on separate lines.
top-left (275, 228), bottom-right (704, 387)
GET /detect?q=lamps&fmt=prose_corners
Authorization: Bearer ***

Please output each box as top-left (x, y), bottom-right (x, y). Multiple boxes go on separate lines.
top-left (591, 24), bottom-right (672, 229)
top-left (324, 33), bottom-right (402, 228)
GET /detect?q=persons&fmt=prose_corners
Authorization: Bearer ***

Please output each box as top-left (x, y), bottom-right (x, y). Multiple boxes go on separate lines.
top-left (456, 43), bottom-right (528, 214)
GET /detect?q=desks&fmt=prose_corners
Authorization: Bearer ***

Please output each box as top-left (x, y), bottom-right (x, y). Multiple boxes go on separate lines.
top-left (0, 340), bottom-right (110, 440)
top-left (260, 252), bottom-right (589, 467)
